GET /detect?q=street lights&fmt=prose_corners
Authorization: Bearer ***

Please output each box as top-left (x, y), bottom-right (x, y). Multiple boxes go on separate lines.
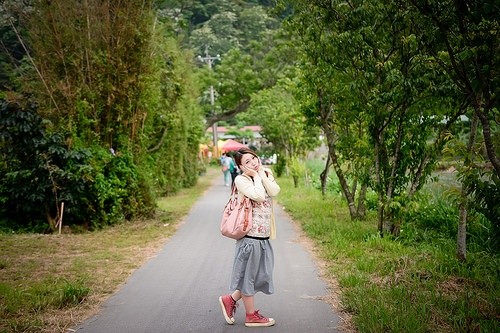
top-left (199, 54), bottom-right (222, 158)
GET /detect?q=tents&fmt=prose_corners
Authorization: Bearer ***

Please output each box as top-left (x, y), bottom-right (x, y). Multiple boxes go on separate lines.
top-left (198, 139), bottom-right (249, 161)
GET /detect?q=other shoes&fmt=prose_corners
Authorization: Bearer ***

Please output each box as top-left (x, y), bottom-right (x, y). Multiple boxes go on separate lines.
top-left (245, 309), bottom-right (275, 327)
top-left (219, 294), bottom-right (239, 325)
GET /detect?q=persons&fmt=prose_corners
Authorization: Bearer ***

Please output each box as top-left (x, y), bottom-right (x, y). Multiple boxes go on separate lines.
top-left (221, 152), bottom-right (239, 188)
top-left (218, 147), bottom-right (280, 327)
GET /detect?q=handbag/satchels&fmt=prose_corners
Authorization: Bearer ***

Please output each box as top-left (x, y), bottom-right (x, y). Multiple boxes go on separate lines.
top-left (222, 166), bottom-right (228, 171)
top-left (220, 174), bottom-right (253, 240)
